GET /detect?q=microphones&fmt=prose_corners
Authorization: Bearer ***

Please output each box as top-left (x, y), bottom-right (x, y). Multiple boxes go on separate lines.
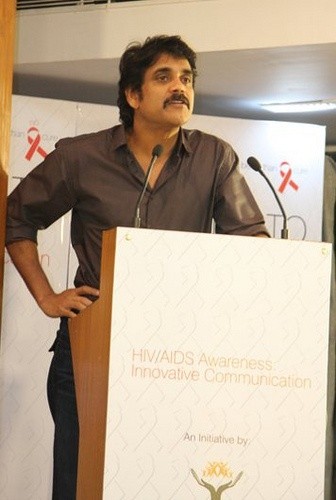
top-left (134, 144), bottom-right (163, 228)
top-left (245, 156), bottom-right (288, 239)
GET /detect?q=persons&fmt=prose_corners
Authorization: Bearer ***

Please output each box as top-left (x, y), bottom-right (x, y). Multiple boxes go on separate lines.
top-left (6, 34), bottom-right (270, 500)
